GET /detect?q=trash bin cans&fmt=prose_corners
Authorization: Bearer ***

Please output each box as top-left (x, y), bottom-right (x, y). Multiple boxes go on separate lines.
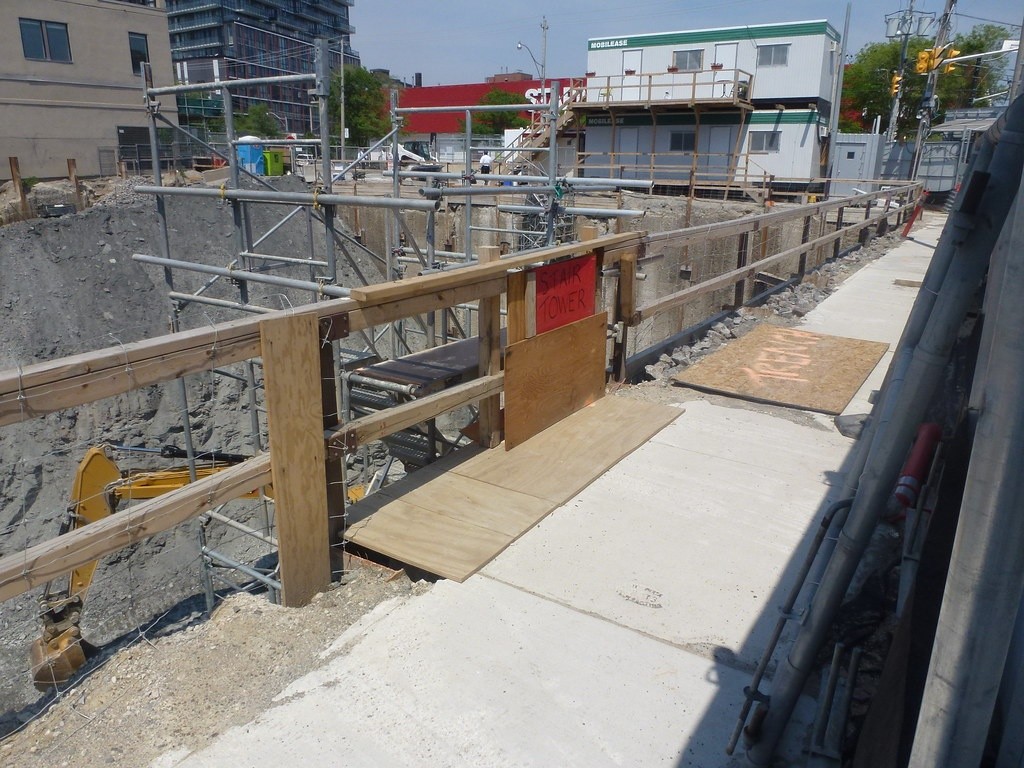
top-left (264, 150), bottom-right (284, 176)
top-left (237, 135), bottom-right (264, 177)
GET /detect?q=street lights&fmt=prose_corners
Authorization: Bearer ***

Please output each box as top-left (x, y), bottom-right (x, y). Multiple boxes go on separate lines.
top-left (516, 17), bottom-right (550, 104)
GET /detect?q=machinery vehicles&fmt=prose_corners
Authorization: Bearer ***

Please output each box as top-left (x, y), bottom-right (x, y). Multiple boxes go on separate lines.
top-left (30, 445), bottom-right (364, 693)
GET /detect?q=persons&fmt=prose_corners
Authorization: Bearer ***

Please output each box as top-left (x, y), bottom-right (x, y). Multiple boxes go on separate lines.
top-left (356, 148), bottom-right (368, 170)
top-left (377, 149), bottom-right (385, 172)
top-left (555, 164), bottom-right (565, 178)
top-left (480, 150), bottom-right (492, 185)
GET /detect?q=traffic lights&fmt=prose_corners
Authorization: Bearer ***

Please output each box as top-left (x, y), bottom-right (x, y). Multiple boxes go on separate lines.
top-left (889, 73), bottom-right (904, 98)
top-left (916, 47), bottom-right (959, 77)
